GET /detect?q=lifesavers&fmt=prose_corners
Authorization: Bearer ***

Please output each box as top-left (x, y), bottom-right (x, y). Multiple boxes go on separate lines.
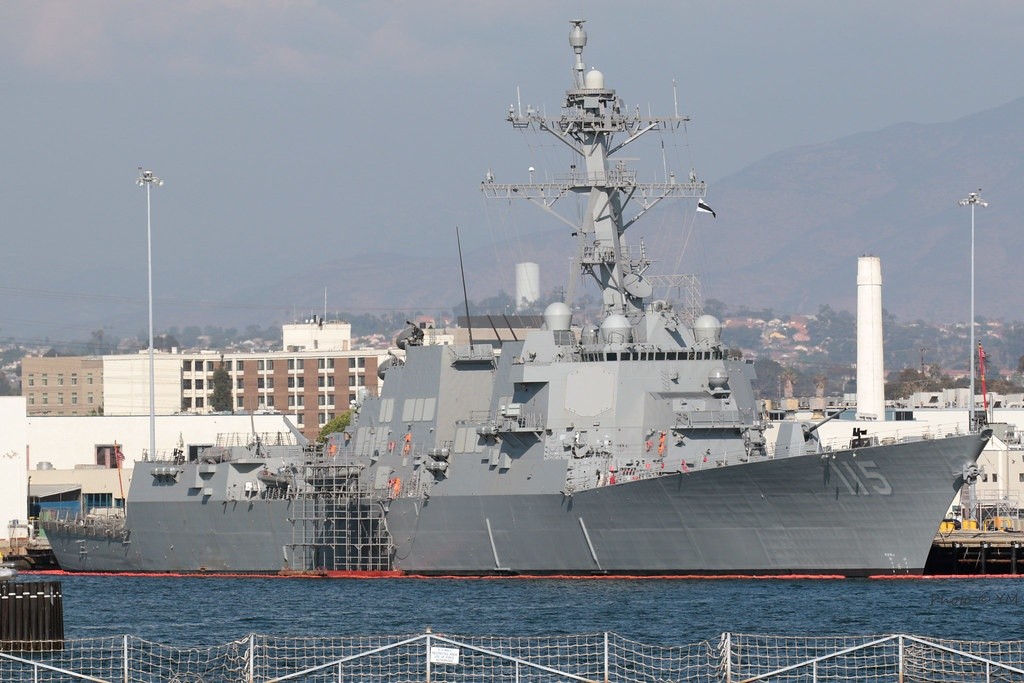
top-left (389, 442), bottom-right (395, 450)
top-left (388, 479), bottom-right (394, 487)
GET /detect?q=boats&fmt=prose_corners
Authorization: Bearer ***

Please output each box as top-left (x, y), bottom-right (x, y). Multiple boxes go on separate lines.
top-left (35, 18), bottom-right (994, 575)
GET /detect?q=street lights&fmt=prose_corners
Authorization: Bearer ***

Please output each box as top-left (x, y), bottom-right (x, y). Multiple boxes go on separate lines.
top-left (135, 169), bottom-right (164, 460)
top-left (955, 193), bottom-right (989, 433)
